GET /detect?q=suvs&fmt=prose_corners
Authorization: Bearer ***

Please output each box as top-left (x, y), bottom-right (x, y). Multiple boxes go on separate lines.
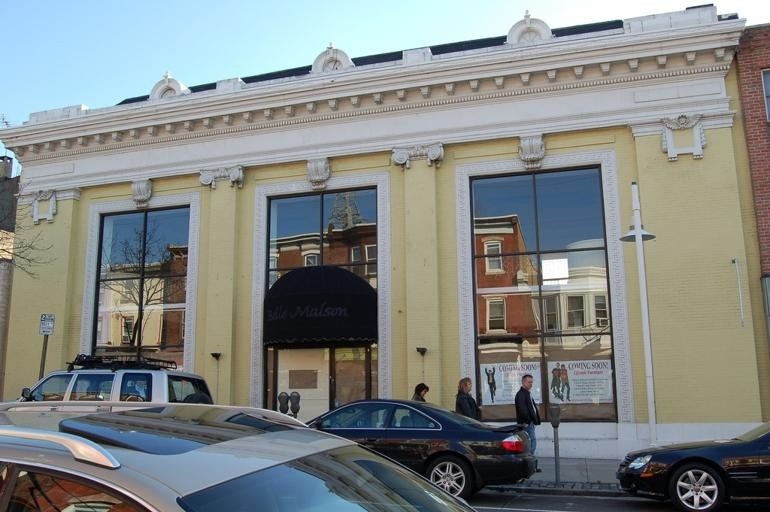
top-left (1, 346), bottom-right (214, 416)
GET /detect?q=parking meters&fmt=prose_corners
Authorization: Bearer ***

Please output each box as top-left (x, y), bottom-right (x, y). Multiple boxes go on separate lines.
top-left (549, 402), bottom-right (562, 483)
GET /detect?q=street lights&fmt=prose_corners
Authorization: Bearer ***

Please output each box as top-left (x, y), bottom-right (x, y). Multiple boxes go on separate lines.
top-left (619, 178), bottom-right (661, 448)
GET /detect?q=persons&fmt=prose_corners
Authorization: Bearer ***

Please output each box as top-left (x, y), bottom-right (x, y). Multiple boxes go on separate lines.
top-left (560, 364), bottom-right (571, 402)
top-left (515, 374), bottom-right (542, 473)
top-left (551, 362), bottom-right (561, 399)
top-left (456, 377), bottom-right (479, 420)
top-left (485, 367), bottom-right (496, 403)
top-left (409, 383), bottom-right (432, 428)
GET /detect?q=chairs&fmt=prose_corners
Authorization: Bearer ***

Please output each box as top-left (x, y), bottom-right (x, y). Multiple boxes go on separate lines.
top-left (400, 415), bottom-right (413, 428)
top-left (379, 411), bottom-right (396, 428)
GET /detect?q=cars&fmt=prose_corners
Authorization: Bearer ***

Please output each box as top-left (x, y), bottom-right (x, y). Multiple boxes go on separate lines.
top-left (304, 397), bottom-right (537, 500)
top-left (1, 400), bottom-right (486, 511)
top-left (616, 420), bottom-right (770, 511)
